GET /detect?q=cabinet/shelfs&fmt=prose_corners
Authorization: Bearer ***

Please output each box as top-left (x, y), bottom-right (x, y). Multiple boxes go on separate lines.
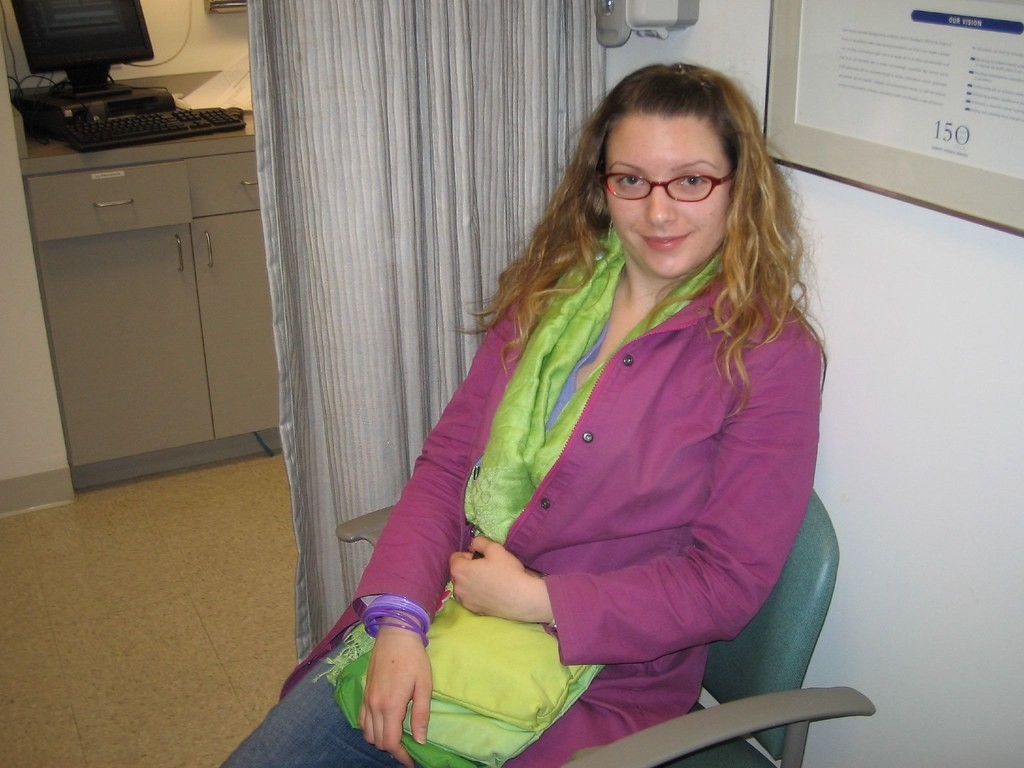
top-left (21, 150), bottom-right (281, 469)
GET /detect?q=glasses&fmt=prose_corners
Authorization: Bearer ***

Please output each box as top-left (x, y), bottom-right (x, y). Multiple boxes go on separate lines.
top-left (601, 171), bottom-right (740, 204)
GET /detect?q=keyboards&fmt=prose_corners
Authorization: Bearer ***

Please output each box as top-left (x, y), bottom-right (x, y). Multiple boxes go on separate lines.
top-left (58, 107), bottom-right (246, 152)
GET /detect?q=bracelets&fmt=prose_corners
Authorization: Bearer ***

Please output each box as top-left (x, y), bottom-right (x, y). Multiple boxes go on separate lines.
top-left (361, 594), bottom-right (431, 648)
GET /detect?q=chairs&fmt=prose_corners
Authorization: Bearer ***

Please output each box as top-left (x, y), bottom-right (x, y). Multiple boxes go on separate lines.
top-left (334, 486), bottom-right (877, 768)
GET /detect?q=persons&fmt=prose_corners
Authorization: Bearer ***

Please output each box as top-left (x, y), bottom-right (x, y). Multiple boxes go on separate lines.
top-left (218, 63), bottom-right (827, 768)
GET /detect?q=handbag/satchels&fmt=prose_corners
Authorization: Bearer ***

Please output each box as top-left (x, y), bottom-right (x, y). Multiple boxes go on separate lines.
top-left (331, 649), bottom-right (488, 766)
top-left (421, 597), bottom-right (591, 733)
top-left (360, 647), bottom-right (606, 768)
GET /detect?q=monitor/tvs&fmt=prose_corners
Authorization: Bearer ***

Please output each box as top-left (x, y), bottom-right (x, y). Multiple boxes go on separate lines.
top-left (10, 0), bottom-right (154, 101)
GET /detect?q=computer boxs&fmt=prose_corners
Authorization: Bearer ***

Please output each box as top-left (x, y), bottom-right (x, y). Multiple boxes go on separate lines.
top-left (18, 87), bottom-right (175, 142)
